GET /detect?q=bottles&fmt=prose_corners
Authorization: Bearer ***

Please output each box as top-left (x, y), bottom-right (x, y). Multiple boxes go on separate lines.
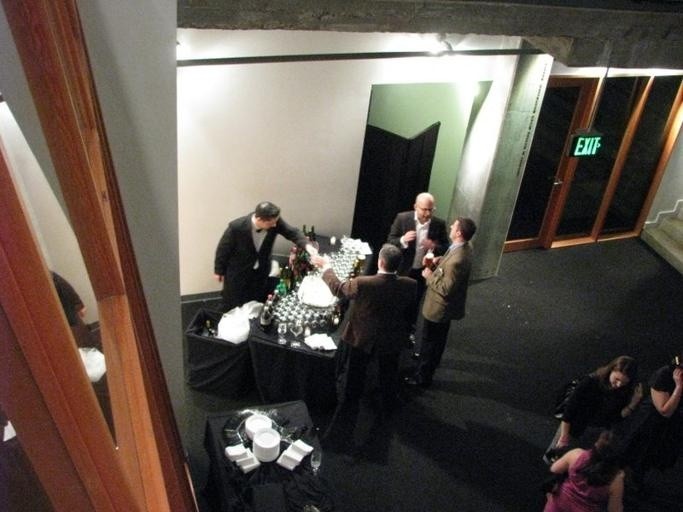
top-left (204, 320), bottom-right (217, 339)
top-left (259, 224), bottom-right (316, 331)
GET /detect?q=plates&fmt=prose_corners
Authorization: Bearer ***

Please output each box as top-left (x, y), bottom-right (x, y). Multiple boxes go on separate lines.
top-left (244, 414), bottom-right (281, 462)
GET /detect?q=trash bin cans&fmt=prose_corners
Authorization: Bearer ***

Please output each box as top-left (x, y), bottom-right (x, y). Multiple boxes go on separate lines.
top-left (185, 307), bottom-right (254, 397)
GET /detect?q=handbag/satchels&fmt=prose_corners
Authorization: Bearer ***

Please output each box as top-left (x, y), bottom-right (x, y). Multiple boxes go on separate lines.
top-left (554, 374), bottom-right (586, 437)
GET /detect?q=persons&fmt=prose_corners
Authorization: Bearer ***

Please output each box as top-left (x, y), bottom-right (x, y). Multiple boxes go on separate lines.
top-left (50, 271), bottom-right (87, 337)
top-left (213, 201), bottom-right (319, 313)
top-left (319, 243), bottom-right (418, 452)
top-left (404, 216), bottom-right (476, 388)
top-left (541, 426), bottom-right (626, 512)
top-left (543, 355), bottom-right (643, 465)
top-left (611, 356), bottom-right (683, 491)
top-left (388, 193), bottom-right (450, 348)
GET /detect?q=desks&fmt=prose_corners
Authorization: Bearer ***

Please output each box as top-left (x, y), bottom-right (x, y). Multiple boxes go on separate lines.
top-left (249, 231), bottom-right (374, 416)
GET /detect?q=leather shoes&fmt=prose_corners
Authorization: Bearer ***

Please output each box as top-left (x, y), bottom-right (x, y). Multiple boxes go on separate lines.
top-left (406, 333), bottom-right (433, 387)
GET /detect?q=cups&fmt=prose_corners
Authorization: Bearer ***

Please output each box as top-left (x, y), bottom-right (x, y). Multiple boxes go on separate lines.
top-left (424, 252), bottom-right (434, 269)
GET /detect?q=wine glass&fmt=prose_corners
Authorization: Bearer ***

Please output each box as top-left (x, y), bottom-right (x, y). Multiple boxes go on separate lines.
top-left (311, 451), bottom-right (321, 473)
top-left (276, 234), bottom-right (366, 348)
